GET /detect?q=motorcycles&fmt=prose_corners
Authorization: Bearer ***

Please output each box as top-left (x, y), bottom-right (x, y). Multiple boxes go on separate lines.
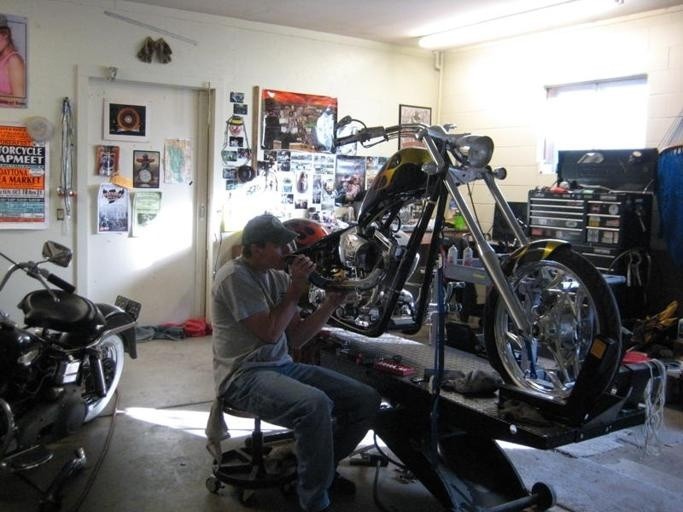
top-left (0, 240), bottom-right (141, 473)
top-left (283, 115), bottom-right (623, 412)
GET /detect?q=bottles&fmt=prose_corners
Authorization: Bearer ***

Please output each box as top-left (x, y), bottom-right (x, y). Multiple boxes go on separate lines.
top-left (453, 209), bottom-right (465, 231)
top-left (448, 244), bottom-right (474, 267)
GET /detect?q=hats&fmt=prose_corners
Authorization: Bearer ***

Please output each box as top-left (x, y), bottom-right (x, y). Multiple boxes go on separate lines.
top-left (243, 214), bottom-right (301, 247)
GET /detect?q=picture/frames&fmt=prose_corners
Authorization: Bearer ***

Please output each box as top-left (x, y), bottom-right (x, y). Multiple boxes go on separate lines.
top-left (398, 104), bottom-right (432, 149)
top-left (1, 15), bottom-right (30, 109)
top-left (253, 86), bottom-right (339, 163)
top-left (104, 100), bottom-right (150, 142)
top-left (0, 122), bottom-right (50, 229)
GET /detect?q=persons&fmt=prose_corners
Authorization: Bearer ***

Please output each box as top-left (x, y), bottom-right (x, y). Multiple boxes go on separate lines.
top-left (0, 14), bottom-right (25, 103)
top-left (211, 214), bottom-right (381, 512)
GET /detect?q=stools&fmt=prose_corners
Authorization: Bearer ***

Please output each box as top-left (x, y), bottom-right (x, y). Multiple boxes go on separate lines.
top-left (205, 406), bottom-right (297, 507)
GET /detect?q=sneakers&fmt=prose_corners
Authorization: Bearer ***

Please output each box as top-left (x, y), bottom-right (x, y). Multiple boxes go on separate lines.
top-left (333, 471), bottom-right (357, 496)
